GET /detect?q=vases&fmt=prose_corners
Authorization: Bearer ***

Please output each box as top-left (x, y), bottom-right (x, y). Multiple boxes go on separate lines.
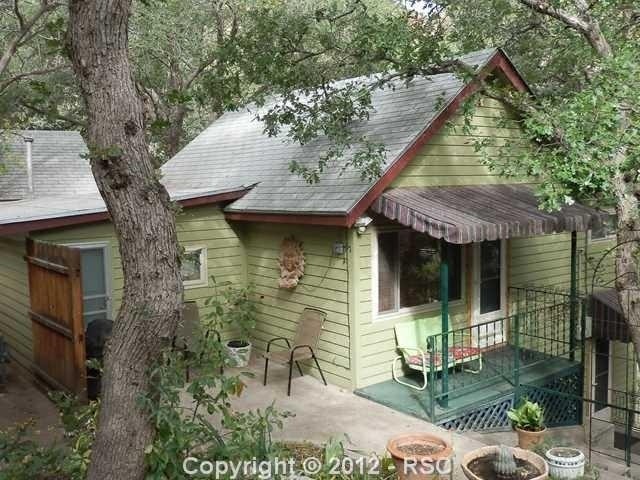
top-left (547, 447), bottom-right (586, 480)
top-left (386, 433), bottom-right (452, 480)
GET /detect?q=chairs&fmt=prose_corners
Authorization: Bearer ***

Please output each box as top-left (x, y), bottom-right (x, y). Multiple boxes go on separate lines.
top-left (262, 306), bottom-right (327, 398)
top-left (167, 299), bottom-right (222, 383)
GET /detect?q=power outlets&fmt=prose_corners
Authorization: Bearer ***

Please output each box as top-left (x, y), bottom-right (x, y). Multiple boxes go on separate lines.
top-left (333, 243), bottom-right (343, 255)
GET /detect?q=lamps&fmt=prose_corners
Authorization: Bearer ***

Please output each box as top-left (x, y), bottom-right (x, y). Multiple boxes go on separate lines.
top-left (354, 217), bottom-right (374, 236)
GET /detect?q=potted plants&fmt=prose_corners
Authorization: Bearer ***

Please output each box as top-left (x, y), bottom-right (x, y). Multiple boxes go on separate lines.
top-left (505, 395), bottom-right (547, 449)
top-left (462, 444), bottom-right (549, 480)
top-left (216, 278), bottom-right (260, 369)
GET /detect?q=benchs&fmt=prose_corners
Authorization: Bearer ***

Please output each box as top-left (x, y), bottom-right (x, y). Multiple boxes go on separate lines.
top-left (392, 314), bottom-right (482, 389)
